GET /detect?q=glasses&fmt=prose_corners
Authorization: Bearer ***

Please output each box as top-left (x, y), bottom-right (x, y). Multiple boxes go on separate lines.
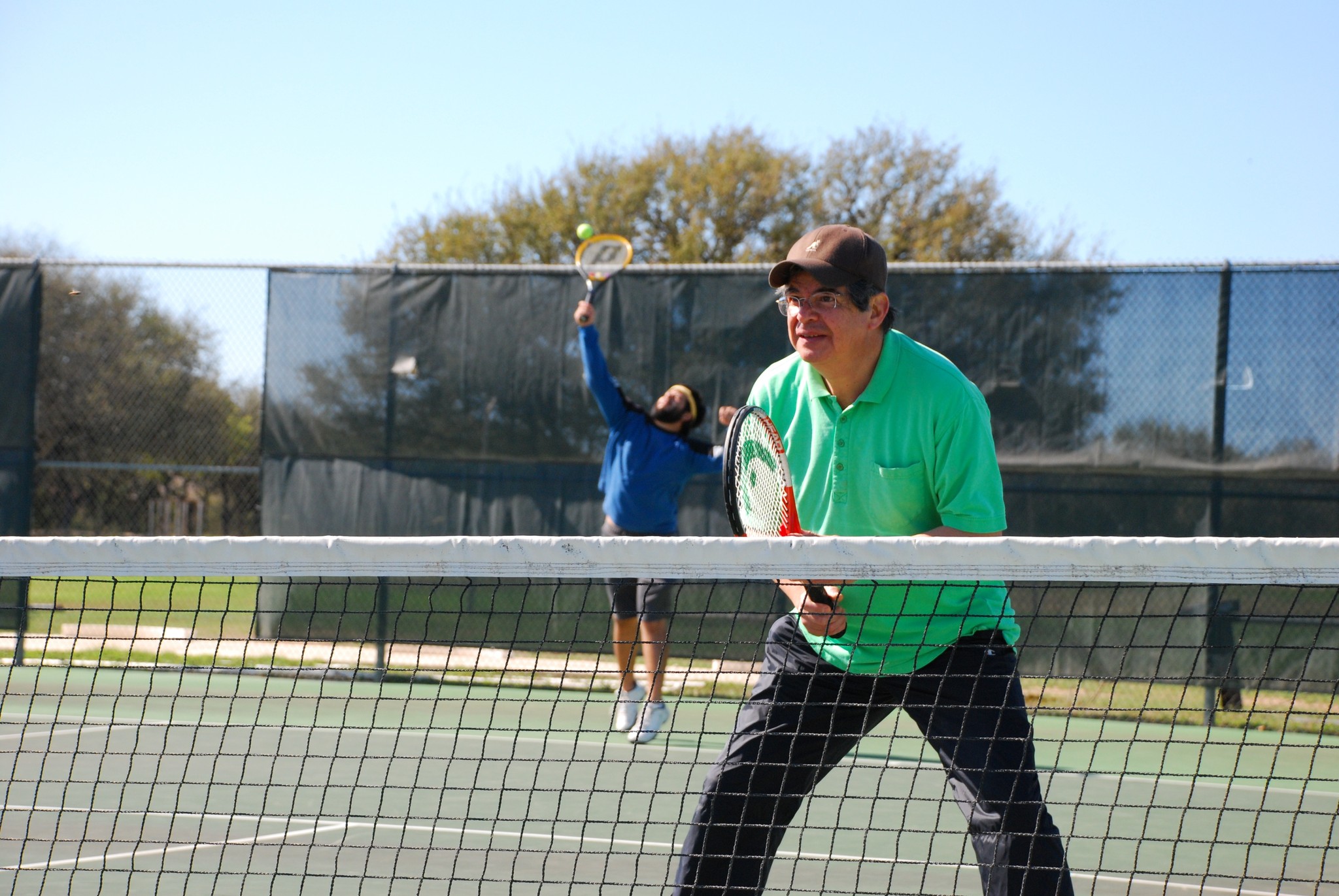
top-left (776, 291), bottom-right (849, 317)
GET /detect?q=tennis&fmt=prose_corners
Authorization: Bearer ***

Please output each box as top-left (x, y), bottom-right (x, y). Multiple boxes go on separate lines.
top-left (576, 223), bottom-right (593, 241)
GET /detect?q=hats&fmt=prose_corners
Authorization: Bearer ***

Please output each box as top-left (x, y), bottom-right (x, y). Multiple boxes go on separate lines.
top-left (769, 224), bottom-right (888, 293)
top-left (671, 384), bottom-right (706, 431)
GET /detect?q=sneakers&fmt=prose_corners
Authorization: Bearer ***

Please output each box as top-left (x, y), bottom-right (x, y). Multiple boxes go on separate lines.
top-left (614, 682), bottom-right (646, 731)
top-left (627, 705), bottom-right (668, 743)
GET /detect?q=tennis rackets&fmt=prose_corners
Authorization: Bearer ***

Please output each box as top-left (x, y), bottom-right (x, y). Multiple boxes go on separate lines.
top-left (722, 406), bottom-right (847, 639)
top-left (575, 234), bottom-right (633, 323)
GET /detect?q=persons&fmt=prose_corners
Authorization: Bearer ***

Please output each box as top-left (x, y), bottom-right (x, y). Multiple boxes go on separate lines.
top-left (674, 225), bottom-right (1074, 896)
top-left (575, 301), bottom-right (740, 745)
